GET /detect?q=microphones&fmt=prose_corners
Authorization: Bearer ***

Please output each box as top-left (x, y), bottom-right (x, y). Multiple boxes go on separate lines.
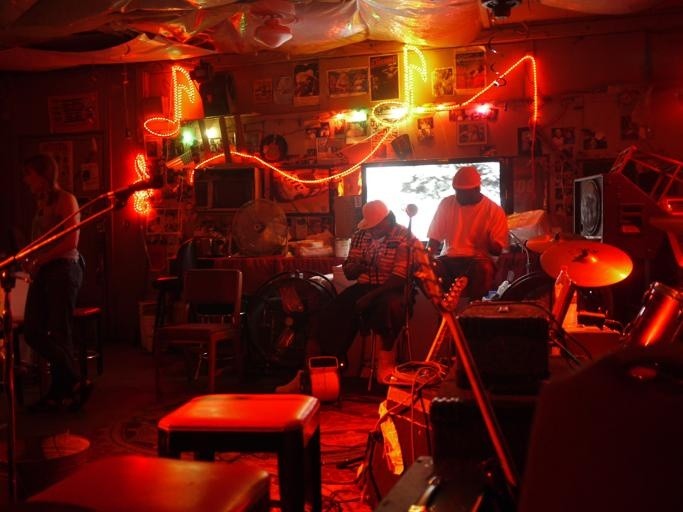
top-left (405, 203), bottom-right (417, 216)
top-left (114, 175), bottom-right (164, 198)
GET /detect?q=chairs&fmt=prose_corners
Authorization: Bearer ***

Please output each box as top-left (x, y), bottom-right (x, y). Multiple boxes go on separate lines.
top-left (154, 265), bottom-right (245, 395)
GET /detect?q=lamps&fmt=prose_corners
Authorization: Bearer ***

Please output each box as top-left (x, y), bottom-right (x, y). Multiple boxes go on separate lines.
top-left (247, 1), bottom-right (299, 51)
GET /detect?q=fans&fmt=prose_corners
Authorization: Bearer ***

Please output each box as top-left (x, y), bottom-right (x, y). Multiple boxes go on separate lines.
top-left (230, 197), bottom-right (288, 265)
top-left (239, 270), bottom-right (339, 376)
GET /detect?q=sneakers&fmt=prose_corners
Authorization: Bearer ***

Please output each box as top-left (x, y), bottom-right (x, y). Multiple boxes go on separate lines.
top-left (65, 381), bottom-right (90, 411)
top-left (275, 370), bottom-right (309, 394)
top-left (377, 340), bottom-right (397, 384)
top-left (28, 394), bottom-right (60, 413)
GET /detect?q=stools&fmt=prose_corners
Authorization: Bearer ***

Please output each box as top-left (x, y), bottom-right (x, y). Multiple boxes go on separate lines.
top-left (192, 305), bottom-right (245, 382)
top-left (359, 316), bottom-right (413, 394)
top-left (21, 453), bottom-right (274, 511)
top-left (153, 391), bottom-right (324, 512)
top-left (67, 302), bottom-right (105, 400)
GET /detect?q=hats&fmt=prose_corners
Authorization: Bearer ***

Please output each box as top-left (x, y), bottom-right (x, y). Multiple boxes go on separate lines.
top-left (357, 200), bottom-right (389, 229)
top-left (453, 166), bottom-right (481, 189)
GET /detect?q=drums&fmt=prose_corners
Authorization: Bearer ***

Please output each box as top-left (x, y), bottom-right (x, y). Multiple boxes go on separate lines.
top-left (622, 282), bottom-right (682, 350)
top-left (566, 333), bottom-right (623, 373)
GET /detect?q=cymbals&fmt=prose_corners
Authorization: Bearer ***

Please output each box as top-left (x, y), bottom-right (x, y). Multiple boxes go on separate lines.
top-left (539, 241), bottom-right (634, 290)
top-left (526, 235), bottom-right (566, 252)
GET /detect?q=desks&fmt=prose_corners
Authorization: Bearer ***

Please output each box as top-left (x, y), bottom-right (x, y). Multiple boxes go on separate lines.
top-left (168, 250), bottom-right (359, 378)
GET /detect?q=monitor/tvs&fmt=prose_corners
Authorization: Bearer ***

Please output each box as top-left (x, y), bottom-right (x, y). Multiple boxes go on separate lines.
top-left (361, 155), bottom-right (509, 250)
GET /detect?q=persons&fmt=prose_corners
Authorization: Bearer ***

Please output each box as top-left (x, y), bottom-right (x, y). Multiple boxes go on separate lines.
top-left (19, 151), bottom-right (93, 413)
top-left (275, 200), bottom-right (422, 393)
top-left (414, 167), bottom-right (508, 311)
